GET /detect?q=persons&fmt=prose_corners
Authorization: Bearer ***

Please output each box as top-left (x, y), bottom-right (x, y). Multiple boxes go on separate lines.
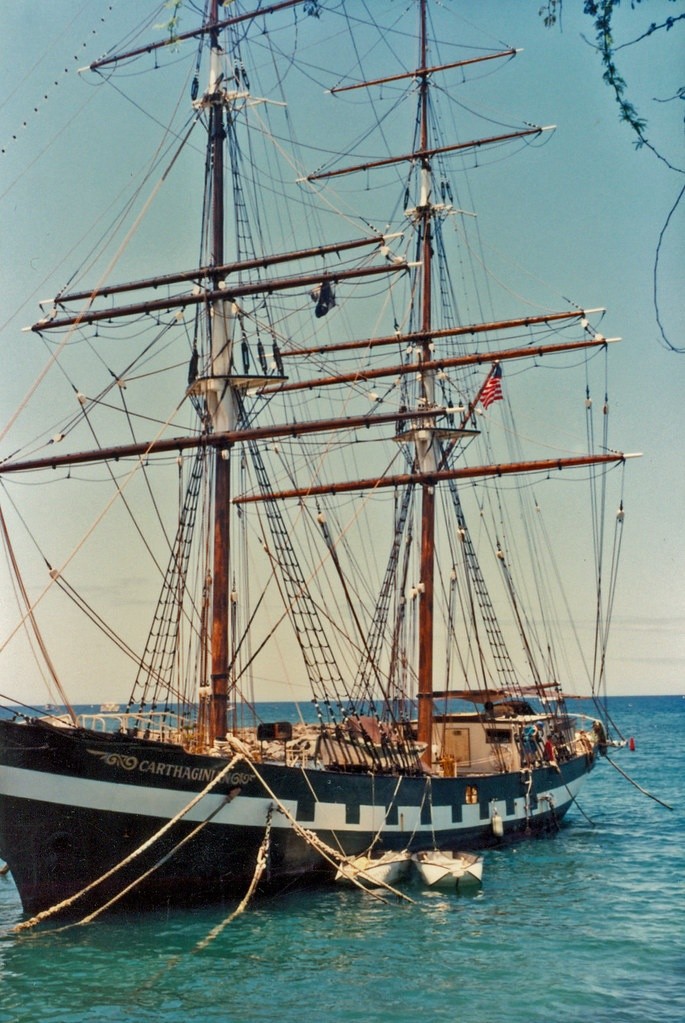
top-left (545, 735), bottom-right (560, 763)
top-left (522, 720), bottom-right (544, 768)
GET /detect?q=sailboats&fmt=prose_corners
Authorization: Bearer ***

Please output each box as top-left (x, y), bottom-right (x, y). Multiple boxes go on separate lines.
top-left (0, 0), bottom-right (646, 921)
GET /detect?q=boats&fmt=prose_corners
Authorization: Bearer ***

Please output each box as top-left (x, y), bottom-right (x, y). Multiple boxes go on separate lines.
top-left (411, 847), bottom-right (483, 888)
top-left (333, 849), bottom-right (413, 888)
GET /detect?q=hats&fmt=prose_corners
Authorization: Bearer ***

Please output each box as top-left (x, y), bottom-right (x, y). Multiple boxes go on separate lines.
top-left (536, 721), bottom-right (544, 728)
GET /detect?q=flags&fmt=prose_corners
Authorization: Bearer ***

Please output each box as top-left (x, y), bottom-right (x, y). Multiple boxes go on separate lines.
top-left (315, 281), bottom-right (337, 318)
top-left (478, 366), bottom-right (505, 411)
